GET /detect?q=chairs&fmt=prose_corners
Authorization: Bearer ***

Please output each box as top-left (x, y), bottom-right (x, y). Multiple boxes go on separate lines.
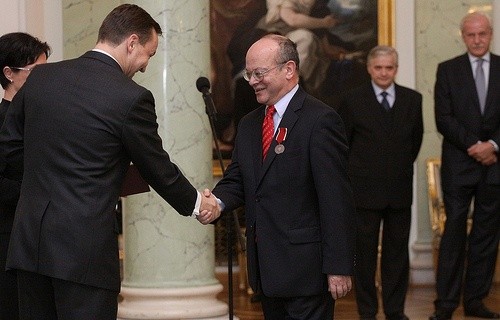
top-left (427, 159), bottom-right (474, 280)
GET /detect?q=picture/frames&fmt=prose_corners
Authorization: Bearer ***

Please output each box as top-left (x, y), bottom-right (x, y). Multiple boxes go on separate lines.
top-left (208, 0), bottom-right (395, 176)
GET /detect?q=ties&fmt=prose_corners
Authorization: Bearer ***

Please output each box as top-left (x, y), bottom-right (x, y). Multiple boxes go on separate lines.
top-left (381, 91), bottom-right (390, 110)
top-left (476, 58), bottom-right (486, 115)
top-left (263, 105), bottom-right (275, 162)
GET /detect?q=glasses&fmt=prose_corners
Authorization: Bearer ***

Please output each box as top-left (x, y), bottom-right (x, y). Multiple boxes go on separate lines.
top-left (8, 67), bottom-right (34, 72)
top-left (242, 61), bottom-right (289, 82)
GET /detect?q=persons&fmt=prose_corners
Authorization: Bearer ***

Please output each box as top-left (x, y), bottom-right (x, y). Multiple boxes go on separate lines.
top-left (196, 34), bottom-right (358, 320)
top-left (335, 45), bottom-right (423, 320)
top-left (428, 12), bottom-right (500, 320)
top-left (1, 32), bottom-right (51, 320)
top-left (0, 3), bottom-right (221, 320)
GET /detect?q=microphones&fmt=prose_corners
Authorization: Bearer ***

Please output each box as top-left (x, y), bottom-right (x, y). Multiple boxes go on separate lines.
top-left (196, 76), bottom-right (217, 115)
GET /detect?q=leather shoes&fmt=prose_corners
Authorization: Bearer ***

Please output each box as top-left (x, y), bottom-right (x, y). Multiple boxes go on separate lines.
top-left (463, 303), bottom-right (500, 319)
top-left (428, 309), bottom-right (453, 320)
